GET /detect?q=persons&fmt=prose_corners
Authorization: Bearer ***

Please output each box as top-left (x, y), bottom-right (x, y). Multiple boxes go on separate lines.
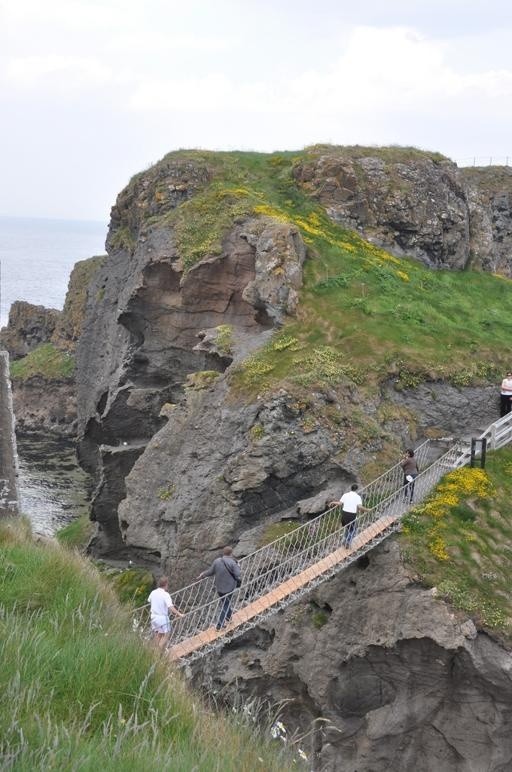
top-left (501, 372), bottom-right (512, 417)
top-left (328, 484), bottom-right (371, 549)
top-left (197, 546), bottom-right (241, 632)
top-left (402, 448), bottom-right (419, 503)
top-left (147, 576), bottom-right (185, 649)
